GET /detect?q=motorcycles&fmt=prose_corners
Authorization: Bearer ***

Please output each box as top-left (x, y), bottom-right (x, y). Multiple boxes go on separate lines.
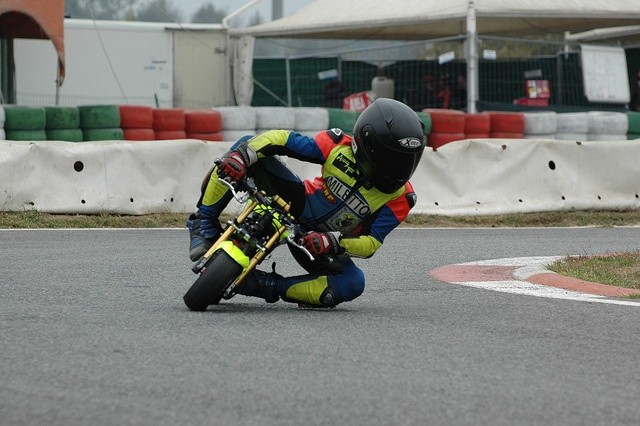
top-left (181, 157), bottom-right (334, 314)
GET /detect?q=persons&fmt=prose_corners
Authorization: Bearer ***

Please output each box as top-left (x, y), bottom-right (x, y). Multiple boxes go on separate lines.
top-left (188, 99), bottom-right (426, 316)
